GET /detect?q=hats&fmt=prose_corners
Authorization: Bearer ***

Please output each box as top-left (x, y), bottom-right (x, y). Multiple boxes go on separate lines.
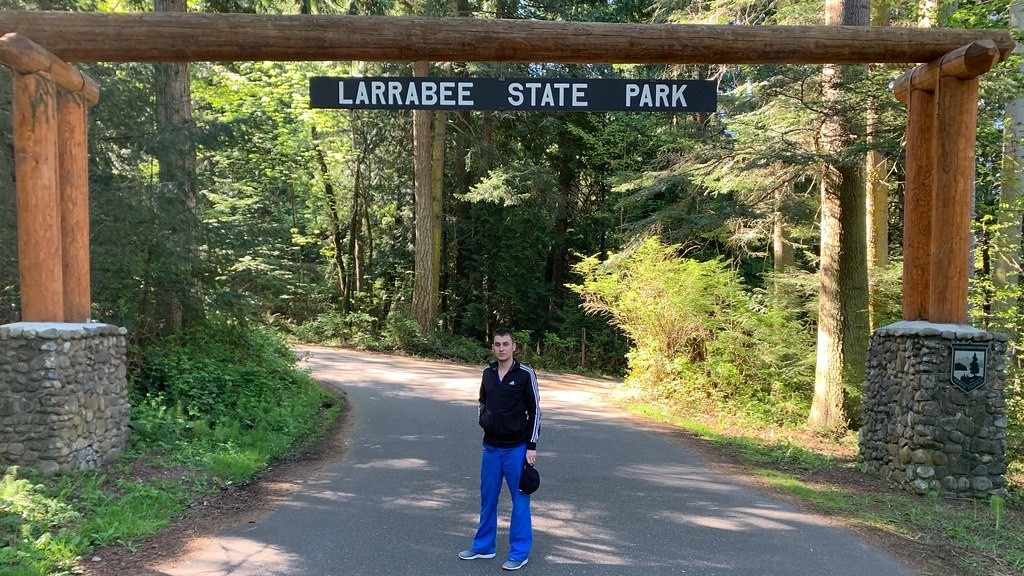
top-left (518, 459), bottom-right (540, 495)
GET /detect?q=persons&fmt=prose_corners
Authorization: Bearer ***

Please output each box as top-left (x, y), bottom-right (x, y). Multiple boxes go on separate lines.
top-left (458, 329), bottom-right (542, 570)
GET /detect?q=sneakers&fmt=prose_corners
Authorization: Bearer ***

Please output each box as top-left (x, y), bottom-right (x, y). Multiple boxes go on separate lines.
top-left (502, 558), bottom-right (528, 570)
top-left (458, 549), bottom-right (496, 560)
